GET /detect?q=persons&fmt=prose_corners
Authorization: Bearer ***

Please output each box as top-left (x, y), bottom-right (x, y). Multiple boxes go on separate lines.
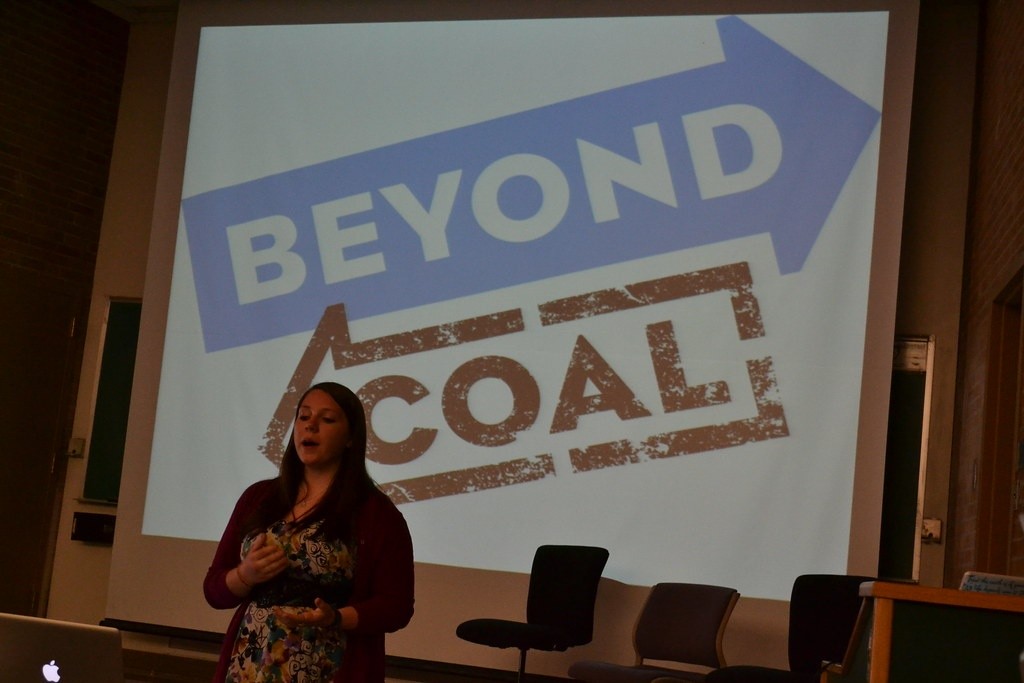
top-left (203, 382), bottom-right (415, 683)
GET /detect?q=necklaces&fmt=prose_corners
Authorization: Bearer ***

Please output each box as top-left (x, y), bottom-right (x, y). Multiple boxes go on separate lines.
top-left (301, 487), bottom-right (327, 507)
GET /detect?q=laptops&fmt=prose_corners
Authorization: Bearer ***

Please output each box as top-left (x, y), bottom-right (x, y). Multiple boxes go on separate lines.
top-left (959, 571), bottom-right (1024, 595)
top-left (0, 613), bottom-right (125, 683)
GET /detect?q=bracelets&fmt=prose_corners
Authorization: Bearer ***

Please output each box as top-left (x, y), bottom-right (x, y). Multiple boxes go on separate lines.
top-left (330, 610), bottom-right (342, 628)
top-left (237, 569), bottom-right (253, 588)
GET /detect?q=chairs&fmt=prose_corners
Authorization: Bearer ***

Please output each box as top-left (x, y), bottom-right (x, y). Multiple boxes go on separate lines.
top-left (456, 545), bottom-right (611, 683)
top-left (705, 574), bottom-right (886, 683)
top-left (569, 582), bottom-right (743, 683)
